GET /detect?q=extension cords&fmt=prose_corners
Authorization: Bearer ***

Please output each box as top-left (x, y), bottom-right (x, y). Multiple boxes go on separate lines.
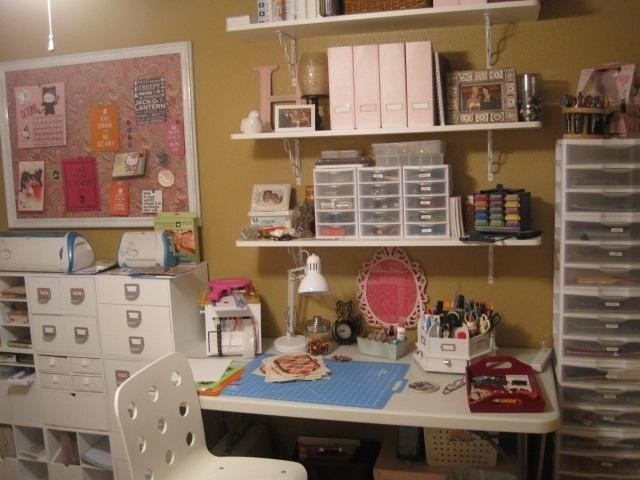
top-left (531, 345), bottom-right (553, 373)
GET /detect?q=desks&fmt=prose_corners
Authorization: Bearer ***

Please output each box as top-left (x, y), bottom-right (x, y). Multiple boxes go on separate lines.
top-left (188, 338), bottom-right (560, 480)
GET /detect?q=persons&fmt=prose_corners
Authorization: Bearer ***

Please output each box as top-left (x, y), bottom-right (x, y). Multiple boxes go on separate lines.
top-left (277, 108), bottom-right (312, 128)
top-left (480, 87), bottom-right (498, 109)
top-left (463, 87), bottom-right (481, 112)
top-left (257, 191), bottom-right (274, 207)
top-left (272, 193), bottom-right (283, 206)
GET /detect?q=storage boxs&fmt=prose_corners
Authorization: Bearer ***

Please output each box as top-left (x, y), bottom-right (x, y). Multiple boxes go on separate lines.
top-left (312, 141), bottom-right (449, 241)
top-left (552, 139), bottom-right (640, 480)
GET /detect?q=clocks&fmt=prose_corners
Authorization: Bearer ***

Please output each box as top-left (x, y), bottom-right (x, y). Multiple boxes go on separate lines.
top-left (332, 300), bottom-right (361, 345)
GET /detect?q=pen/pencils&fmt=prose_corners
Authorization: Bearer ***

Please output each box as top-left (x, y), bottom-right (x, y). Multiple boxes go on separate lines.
top-left (423, 293), bottom-right (495, 338)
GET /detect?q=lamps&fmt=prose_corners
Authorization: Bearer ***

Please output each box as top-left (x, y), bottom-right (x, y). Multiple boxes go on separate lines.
top-left (275, 252), bottom-right (330, 355)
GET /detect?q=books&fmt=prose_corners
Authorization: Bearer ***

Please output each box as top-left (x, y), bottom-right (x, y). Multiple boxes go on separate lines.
top-left (102, 266), bottom-right (194, 279)
top-left (8, 336), bottom-right (31, 348)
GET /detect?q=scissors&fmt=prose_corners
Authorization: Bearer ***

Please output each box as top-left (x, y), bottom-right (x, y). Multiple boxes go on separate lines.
top-left (464, 310), bottom-right (501, 333)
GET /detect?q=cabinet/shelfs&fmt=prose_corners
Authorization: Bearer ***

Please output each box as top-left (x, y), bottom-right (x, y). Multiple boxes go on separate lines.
top-left (0, 261), bottom-right (213, 480)
top-left (225, 0), bottom-right (543, 283)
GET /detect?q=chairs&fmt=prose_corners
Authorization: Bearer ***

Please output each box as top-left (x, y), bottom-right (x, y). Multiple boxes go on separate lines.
top-left (115, 352), bottom-right (307, 480)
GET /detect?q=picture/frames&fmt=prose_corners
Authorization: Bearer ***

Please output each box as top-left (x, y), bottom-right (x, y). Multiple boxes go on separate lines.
top-left (251, 183), bottom-right (290, 210)
top-left (444, 69), bottom-right (518, 123)
top-left (273, 103), bottom-right (316, 132)
